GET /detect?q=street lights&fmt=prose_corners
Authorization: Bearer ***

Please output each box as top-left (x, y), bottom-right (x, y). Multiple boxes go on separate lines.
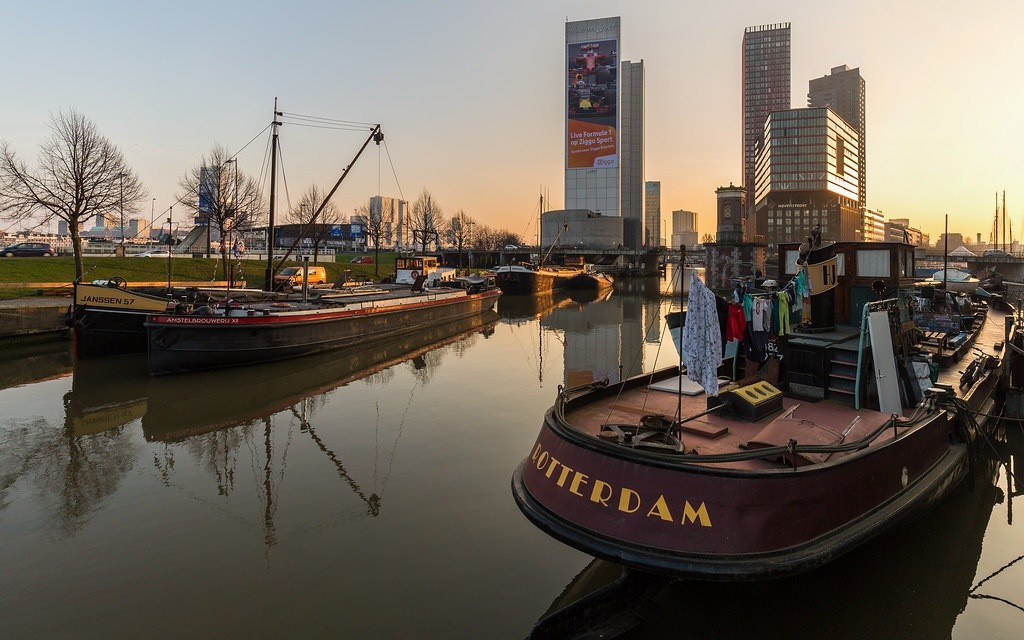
top-left (299, 203), bottom-right (306, 262)
top-left (149, 197), bottom-right (156, 254)
top-left (244, 195), bottom-right (252, 254)
top-left (118, 172), bottom-right (128, 258)
top-left (225, 157), bottom-right (238, 261)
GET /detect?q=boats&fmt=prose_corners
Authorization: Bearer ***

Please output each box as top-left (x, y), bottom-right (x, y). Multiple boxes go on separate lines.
top-left (572, 269), bottom-right (615, 292)
top-left (492, 192), bottom-right (597, 297)
top-left (510, 220), bottom-right (1024, 595)
top-left (60, 354), bottom-right (148, 443)
top-left (140, 309), bottom-right (502, 547)
top-left (54, 96), bottom-right (503, 381)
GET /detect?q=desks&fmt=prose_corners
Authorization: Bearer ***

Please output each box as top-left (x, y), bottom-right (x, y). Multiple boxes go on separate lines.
top-left (925, 332), bottom-right (947, 356)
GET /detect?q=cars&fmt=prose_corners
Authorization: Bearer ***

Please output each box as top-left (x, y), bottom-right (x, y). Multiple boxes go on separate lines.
top-left (504, 244), bottom-right (519, 251)
top-left (0, 242), bottom-right (54, 258)
